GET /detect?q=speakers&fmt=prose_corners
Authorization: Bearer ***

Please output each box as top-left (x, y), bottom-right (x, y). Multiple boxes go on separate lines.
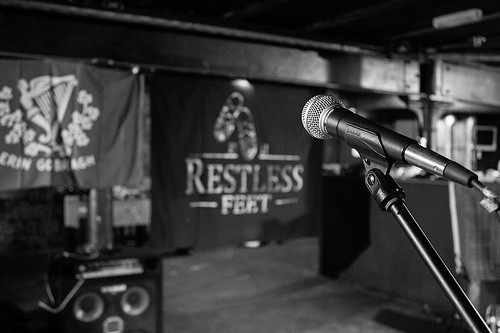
top-left (51, 247), bottom-right (163, 333)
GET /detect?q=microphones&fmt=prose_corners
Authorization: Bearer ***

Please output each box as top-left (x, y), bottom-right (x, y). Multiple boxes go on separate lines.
top-left (301, 93), bottom-right (477, 191)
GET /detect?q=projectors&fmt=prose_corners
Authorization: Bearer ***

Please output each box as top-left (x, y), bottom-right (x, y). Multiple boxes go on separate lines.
top-left (432, 8), bottom-right (483, 30)
